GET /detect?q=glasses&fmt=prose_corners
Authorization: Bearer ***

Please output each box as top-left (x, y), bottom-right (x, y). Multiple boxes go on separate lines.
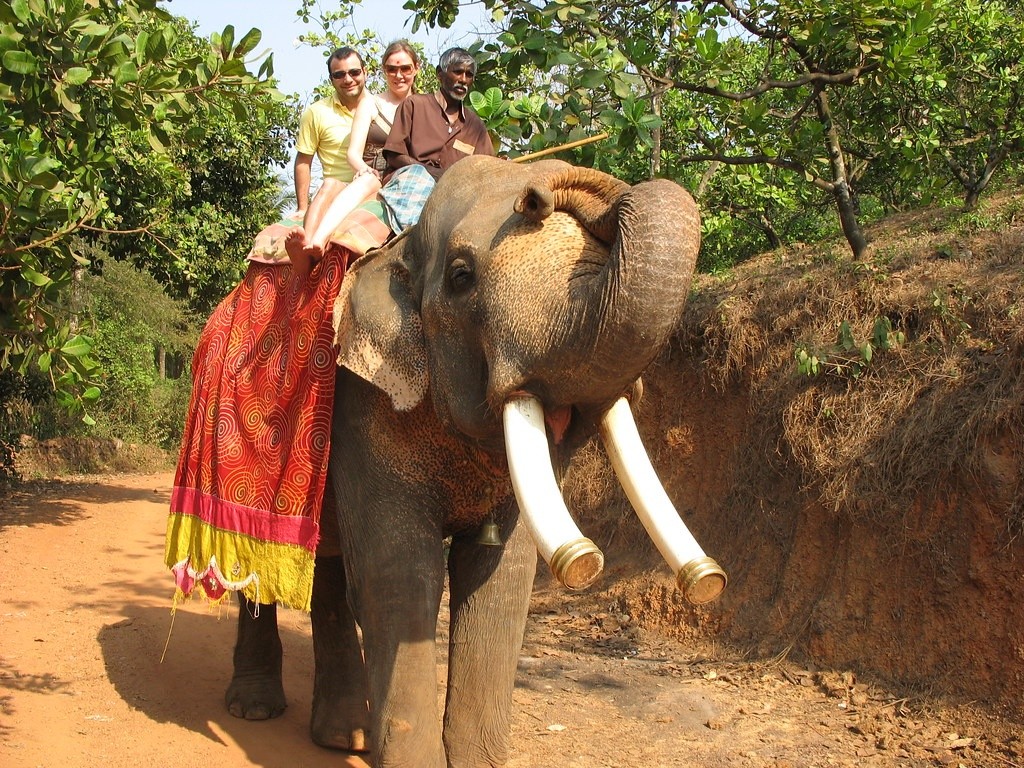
top-left (329, 69), bottom-right (363, 79)
top-left (382, 64), bottom-right (418, 77)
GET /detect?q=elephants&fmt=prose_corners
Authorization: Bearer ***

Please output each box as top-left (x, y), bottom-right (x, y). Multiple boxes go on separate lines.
top-left (224, 154), bottom-right (726, 768)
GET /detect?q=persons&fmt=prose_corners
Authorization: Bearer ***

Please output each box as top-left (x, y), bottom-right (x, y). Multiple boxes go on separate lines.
top-left (283, 41), bottom-right (498, 276)
